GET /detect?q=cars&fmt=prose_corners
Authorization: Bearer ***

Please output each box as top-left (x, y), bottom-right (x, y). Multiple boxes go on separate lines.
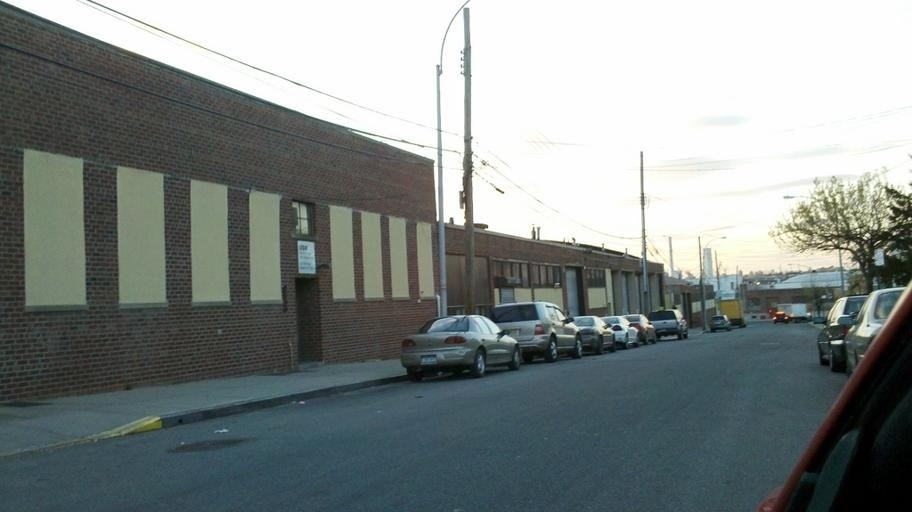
top-left (400, 301), bottom-right (688, 382)
top-left (814, 287), bottom-right (907, 378)
top-left (773, 311), bottom-right (789, 324)
top-left (710, 315), bottom-right (730, 332)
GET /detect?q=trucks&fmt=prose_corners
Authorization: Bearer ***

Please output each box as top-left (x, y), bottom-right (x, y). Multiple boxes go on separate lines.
top-left (719, 299), bottom-right (743, 327)
top-left (768, 303), bottom-right (808, 322)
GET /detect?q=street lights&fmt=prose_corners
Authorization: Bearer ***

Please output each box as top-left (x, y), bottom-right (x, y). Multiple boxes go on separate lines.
top-left (703, 234), bottom-right (727, 330)
top-left (784, 196), bottom-right (844, 295)
